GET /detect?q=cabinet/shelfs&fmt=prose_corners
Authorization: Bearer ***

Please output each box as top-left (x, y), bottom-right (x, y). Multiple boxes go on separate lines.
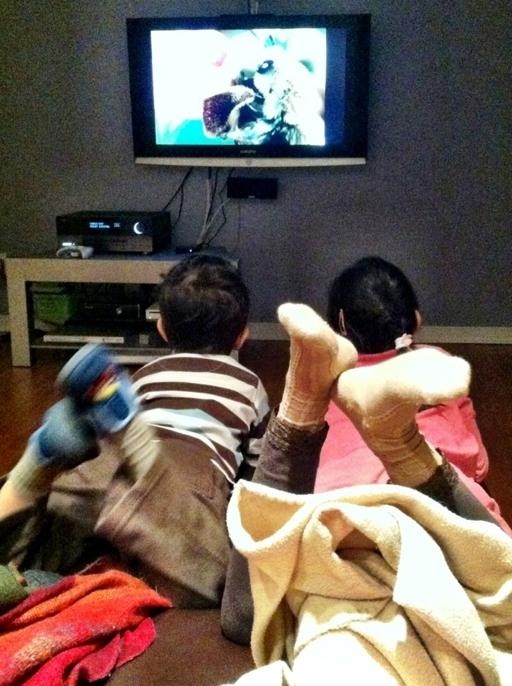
top-left (5, 248), bottom-right (240, 366)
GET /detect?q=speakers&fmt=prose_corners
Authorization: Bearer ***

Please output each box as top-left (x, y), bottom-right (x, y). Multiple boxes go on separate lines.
top-left (226, 177), bottom-right (278, 200)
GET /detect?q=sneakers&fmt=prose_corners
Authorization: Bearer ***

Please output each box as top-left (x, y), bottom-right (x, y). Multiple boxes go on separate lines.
top-left (54, 343), bottom-right (142, 436)
top-left (39, 398), bottom-right (102, 471)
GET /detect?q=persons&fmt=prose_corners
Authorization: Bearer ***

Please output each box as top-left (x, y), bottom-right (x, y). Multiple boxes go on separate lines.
top-left (0, 249), bottom-right (277, 613)
top-left (215, 253), bottom-right (511, 651)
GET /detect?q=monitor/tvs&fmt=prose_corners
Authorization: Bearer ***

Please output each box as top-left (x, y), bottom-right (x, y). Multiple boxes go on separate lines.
top-left (124, 12), bottom-right (373, 169)
